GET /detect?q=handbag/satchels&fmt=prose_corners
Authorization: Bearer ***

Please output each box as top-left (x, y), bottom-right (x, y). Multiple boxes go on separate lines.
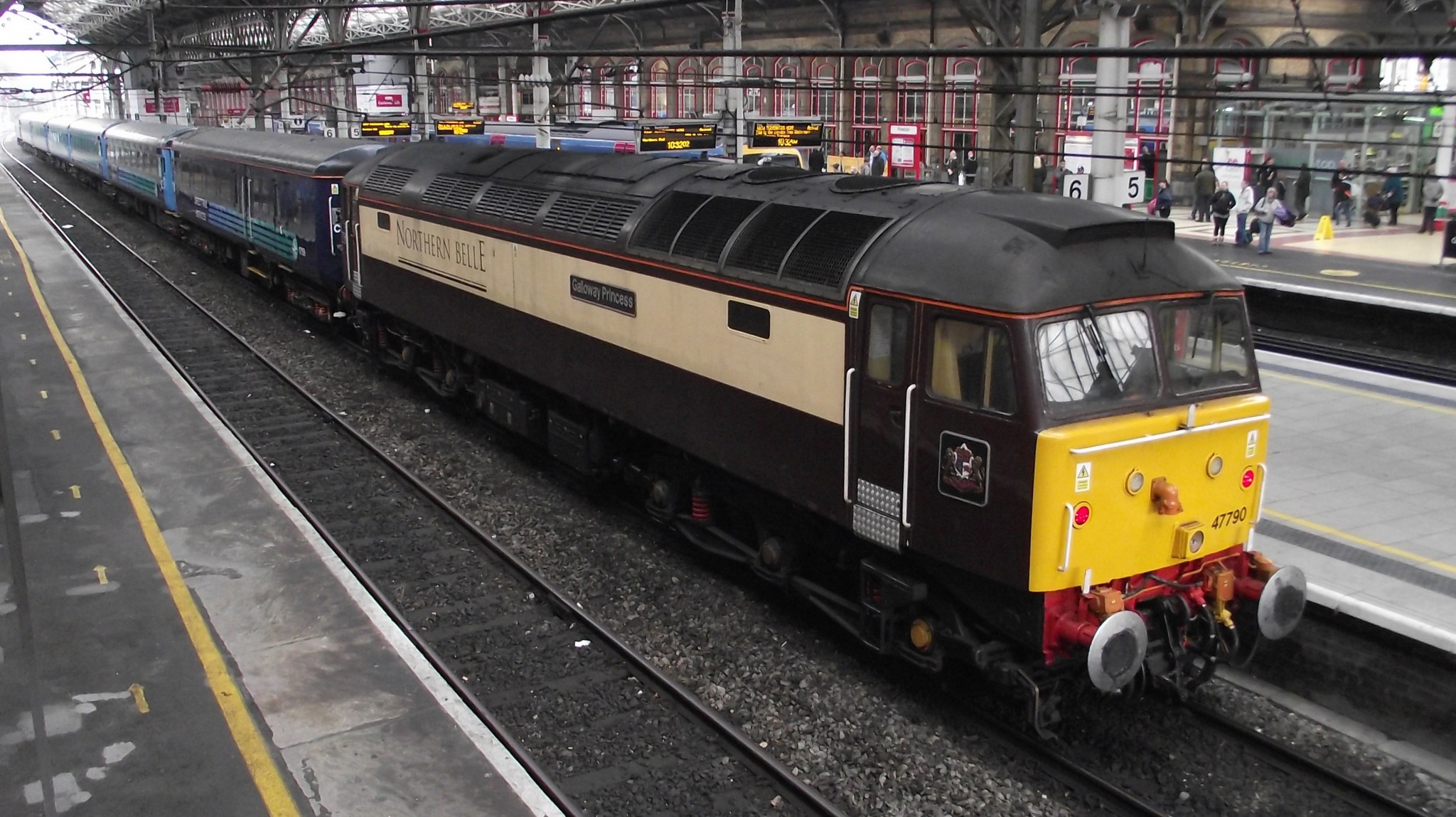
top-left (1250, 219), bottom-right (1261, 233)
top-left (1367, 196), bottom-right (1383, 210)
top-left (958, 173), bottom-right (966, 186)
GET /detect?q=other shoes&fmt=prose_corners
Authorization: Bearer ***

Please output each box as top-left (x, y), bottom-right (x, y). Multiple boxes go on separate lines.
top-left (1195, 219), bottom-right (1202, 222)
top-left (1258, 250), bottom-right (1272, 255)
top-left (1387, 222), bottom-right (1397, 225)
top-left (1207, 219), bottom-right (1211, 222)
top-left (1234, 240), bottom-right (1249, 246)
top-left (1419, 228), bottom-right (1427, 233)
top-left (1297, 213), bottom-right (1309, 221)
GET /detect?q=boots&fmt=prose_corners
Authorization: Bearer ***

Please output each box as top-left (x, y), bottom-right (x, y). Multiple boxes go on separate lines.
top-left (1218, 236), bottom-right (1224, 244)
top-left (1212, 236), bottom-right (1218, 244)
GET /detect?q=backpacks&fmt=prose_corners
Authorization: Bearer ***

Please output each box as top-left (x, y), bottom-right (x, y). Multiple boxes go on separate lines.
top-left (1342, 181), bottom-right (1361, 196)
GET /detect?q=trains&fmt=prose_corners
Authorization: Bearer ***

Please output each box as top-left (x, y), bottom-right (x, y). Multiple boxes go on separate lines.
top-left (14, 110), bottom-right (1309, 756)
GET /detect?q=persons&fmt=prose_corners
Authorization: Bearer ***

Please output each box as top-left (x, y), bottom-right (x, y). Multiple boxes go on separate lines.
top-left (1139, 145), bottom-right (1444, 254)
top-left (810, 144), bottom-right (1084, 196)
